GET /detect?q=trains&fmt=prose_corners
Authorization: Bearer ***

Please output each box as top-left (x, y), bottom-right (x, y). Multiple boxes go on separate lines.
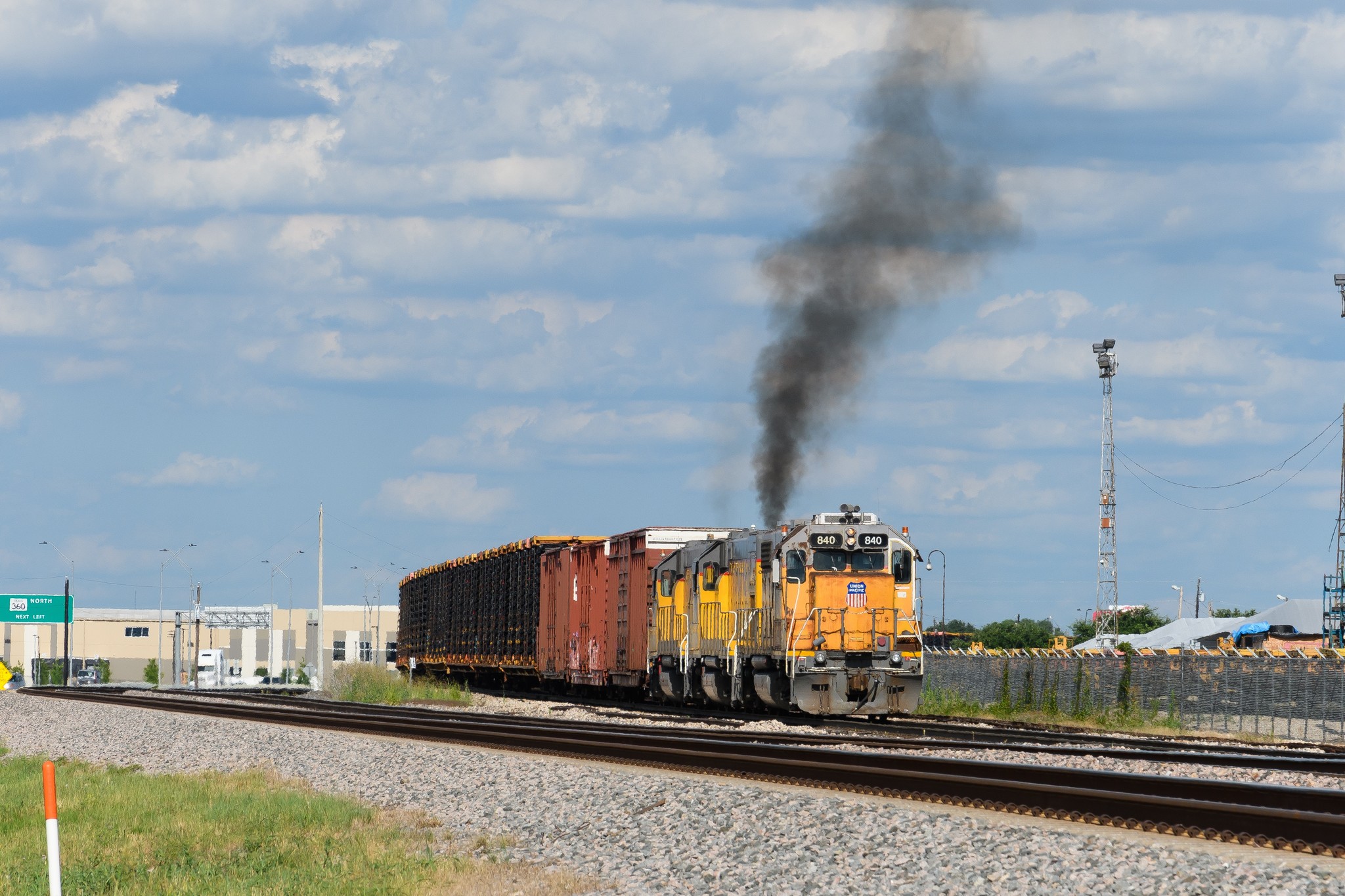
top-left (395, 501), bottom-right (926, 722)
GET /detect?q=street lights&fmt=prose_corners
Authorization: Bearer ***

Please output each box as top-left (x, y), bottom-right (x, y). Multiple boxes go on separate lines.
top-left (158, 543), bottom-right (197, 685)
top-left (33, 635), bottom-right (36, 686)
top-left (39, 541), bottom-right (74, 686)
top-left (350, 566), bottom-right (408, 664)
top-left (926, 549), bottom-right (946, 650)
top-left (363, 562), bottom-right (395, 664)
top-left (1171, 585), bottom-right (1183, 619)
top-left (262, 560), bottom-right (293, 684)
top-left (35, 636), bottom-right (40, 685)
top-left (269, 550), bottom-right (305, 684)
top-left (159, 548), bottom-right (193, 687)
